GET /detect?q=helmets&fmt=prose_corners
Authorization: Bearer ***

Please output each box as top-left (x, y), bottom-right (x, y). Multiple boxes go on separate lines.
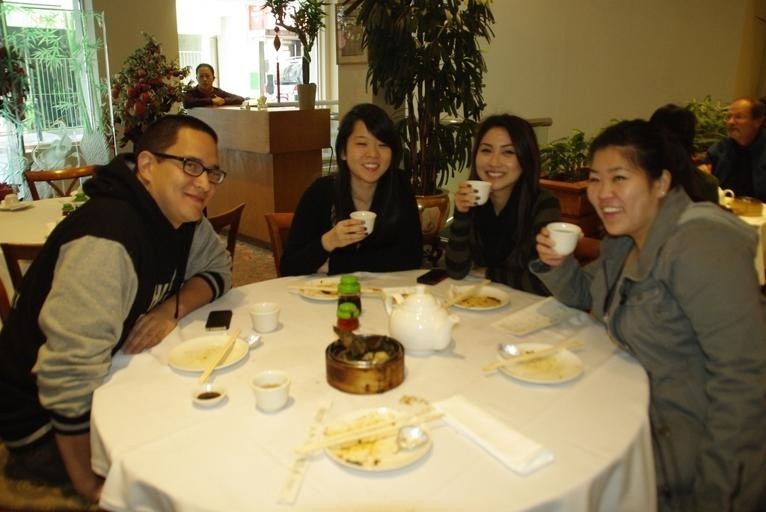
top-left (319, 408), bottom-right (436, 473)
top-left (494, 342), bottom-right (584, 385)
top-left (441, 283), bottom-right (511, 312)
top-left (190, 388), bottom-right (228, 410)
top-left (381, 285), bottom-right (430, 318)
top-left (0, 201), bottom-right (33, 211)
top-left (168, 332), bottom-right (249, 372)
top-left (297, 276), bottom-right (365, 302)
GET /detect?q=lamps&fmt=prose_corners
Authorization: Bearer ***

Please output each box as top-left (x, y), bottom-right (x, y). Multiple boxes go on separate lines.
top-left (0, 242), bottom-right (46, 290)
top-left (1, 441), bottom-right (105, 511)
top-left (573, 239), bottom-right (602, 267)
top-left (263, 211), bottom-right (296, 277)
top-left (207, 201), bottom-right (246, 272)
top-left (22, 165), bottom-right (101, 202)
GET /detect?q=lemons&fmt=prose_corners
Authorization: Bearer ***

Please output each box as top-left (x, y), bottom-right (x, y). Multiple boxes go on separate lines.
top-left (384, 283), bottom-right (460, 355)
top-left (718, 186), bottom-right (735, 208)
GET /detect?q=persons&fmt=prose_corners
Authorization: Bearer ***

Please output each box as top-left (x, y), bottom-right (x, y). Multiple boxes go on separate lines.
top-left (445, 113), bottom-right (561, 296)
top-left (279, 103), bottom-right (421, 277)
top-left (182, 63), bottom-right (245, 108)
top-left (0, 113), bottom-right (234, 502)
top-left (650, 102), bottom-right (720, 206)
top-left (706, 97), bottom-right (766, 203)
top-left (527, 118), bottom-right (766, 512)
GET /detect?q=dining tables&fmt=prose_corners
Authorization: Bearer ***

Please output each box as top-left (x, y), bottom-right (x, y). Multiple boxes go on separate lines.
top-left (260, 0), bottom-right (332, 110)
top-left (336, 0), bottom-right (496, 268)
top-left (538, 127), bottom-right (599, 235)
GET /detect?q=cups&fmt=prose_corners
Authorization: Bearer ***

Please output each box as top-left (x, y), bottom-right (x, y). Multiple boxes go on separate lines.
top-left (725, 113), bottom-right (754, 120)
top-left (135, 149), bottom-right (227, 185)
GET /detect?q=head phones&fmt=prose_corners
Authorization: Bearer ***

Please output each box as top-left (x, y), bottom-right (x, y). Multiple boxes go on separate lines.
top-left (479, 339), bottom-right (583, 373)
top-left (191, 327), bottom-right (243, 385)
top-left (287, 282), bottom-right (380, 294)
top-left (296, 407), bottom-right (442, 454)
top-left (443, 278), bottom-right (491, 309)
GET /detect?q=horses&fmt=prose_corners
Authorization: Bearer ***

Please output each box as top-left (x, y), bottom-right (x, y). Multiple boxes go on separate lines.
top-left (206, 309), bottom-right (230, 327)
top-left (416, 267), bottom-right (449, 286)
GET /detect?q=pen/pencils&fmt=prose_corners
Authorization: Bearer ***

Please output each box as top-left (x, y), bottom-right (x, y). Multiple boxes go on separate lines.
top-left (275, 58), bottom-right (302, 100)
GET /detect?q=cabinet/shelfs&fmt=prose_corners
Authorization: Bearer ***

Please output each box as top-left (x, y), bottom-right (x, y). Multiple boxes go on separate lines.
top-left (337, 302), bottom-right (360, 331)
top-left (338, 275), bottom-right (362, 316)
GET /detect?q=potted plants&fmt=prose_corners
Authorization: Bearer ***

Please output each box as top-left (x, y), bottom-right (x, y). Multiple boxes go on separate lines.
top-left (333, 1), bottom-right (369, 67)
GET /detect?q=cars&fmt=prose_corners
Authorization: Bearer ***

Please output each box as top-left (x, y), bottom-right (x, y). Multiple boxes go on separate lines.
top-left (464, 180), bottom-right (493, 208)
top-left (349, 210), bottom-right (377, 236)
top-left (252, 370), bottom-right (291, 411)
top-left (249, 301), bottom-right (282, 334)
top-left (3, 194), bottom-right (18, 207)
top-left (540, 221), bottom-right (581, 259)
